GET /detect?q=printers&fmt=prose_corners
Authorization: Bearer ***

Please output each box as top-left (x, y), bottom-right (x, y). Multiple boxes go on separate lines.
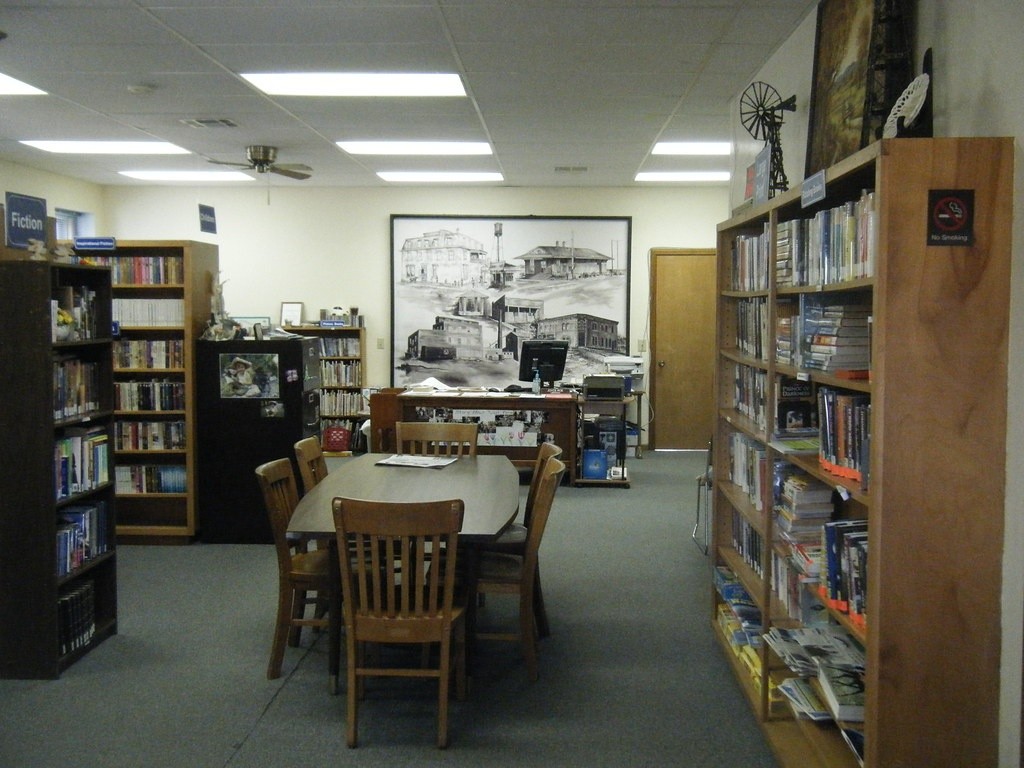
top-left (582, 357), bottom-right (647, 402)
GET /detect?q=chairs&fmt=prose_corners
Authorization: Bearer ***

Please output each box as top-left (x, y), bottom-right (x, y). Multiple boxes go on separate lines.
top-left (425, 457), bottom-right (567, 682)
top-left (458, 442), bottom-right (563, 637)
top-left (396, 421), bottom-right (478, 459)
top-left (331, 495), bottom-right (467, 749)
top-left (294, 435), bottom-right (401, 633)
top-left (256, 458), bottom-right (387, 680)
top-left (692, 435), bottom-right (713, 556)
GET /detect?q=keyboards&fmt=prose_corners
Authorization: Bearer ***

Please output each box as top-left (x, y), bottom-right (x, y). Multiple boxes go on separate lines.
top-left (504, 387), bottom-right (532, 392)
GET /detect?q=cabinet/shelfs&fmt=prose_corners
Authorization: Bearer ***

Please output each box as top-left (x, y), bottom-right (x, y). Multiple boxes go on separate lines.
top-left (624, 392), bottom-right (646, 459)
top-left (576, 395), bottom-right (632, 489)
top-left (0, 260), bottom-right (118, 680)
top-left (55, 239), bottom-right (219, 547)
top-left (196, 337), bottom-right (322, 546)
top-left (282, 327), bottom-right (367, 458)
top-left (713, 136), bottom-right (1013, 768)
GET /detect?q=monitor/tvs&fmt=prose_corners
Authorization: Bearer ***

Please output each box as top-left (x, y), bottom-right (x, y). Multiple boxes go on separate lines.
top-left (519, 340), bottom-right (569, 388)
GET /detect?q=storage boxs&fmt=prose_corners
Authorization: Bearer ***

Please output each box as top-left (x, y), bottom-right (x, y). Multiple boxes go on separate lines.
top-left (626, 428), bottom-right (639, 458)
top-left (718, 604), bottom-right (786, 712)
top-left (582, 448), bottom-right (608, 480)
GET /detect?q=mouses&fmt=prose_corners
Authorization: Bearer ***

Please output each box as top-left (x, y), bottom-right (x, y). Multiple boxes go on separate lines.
top-left (489, 388), bottom-right (499, 392)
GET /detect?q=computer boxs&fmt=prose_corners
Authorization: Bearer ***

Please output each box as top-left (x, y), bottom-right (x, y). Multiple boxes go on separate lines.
top-left (593, 416), bottom-right (623, 470)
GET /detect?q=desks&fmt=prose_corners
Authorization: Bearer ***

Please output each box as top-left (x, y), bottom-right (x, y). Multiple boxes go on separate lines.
top-left (284, 453), bottom-right (520, 649)
top-left (369, 387), bottom-right (576, 489)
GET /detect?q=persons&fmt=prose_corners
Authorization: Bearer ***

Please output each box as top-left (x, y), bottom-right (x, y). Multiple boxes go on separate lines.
top-left (229, 357), bottom-right (261, 397)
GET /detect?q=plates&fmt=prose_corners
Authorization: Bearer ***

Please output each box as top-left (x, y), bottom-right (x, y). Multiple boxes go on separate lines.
top-left (882, 72), bottom-right (930, 139)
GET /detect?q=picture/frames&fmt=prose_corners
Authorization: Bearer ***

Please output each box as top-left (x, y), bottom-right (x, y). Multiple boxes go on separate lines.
top-left (280, 302), bottom-right (304, 327)
top-left (804, 0), bottom-right (894, 179)
top-left (390, 213), bottom-right (632, 391)
top-left (229, 316), bottom-right (270, 328)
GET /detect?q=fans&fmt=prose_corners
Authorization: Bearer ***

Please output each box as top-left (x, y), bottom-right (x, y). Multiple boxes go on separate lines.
top-left (207, 146), bottom-right (314, 181)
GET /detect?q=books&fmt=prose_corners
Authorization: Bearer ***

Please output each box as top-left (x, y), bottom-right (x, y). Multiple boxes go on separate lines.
top-left (734, 365), bottom-right (870, 491)
top-left (54, 424), bottom-right (109, 500)
top-left (114, 379), bottom-right (185, 411)
top-left (56, 584), bottom-right (96, 654)
top-left (734, 293), bottom-right (874, 385)
top-left (729, 189), bottom-right (876, 291)
top-left (56, 501), bottom-right (107, 575)
top-left (116, 498), bottom-right (188, 527)
top-left (712, 430), bottom-right (868, 768)
top-left (413, 386), bottom-right (432, 392)
top-left (54, 360), bottom-right (100, 420)
top-left (114, 421), bottom-right (186, 450)
top-left (71, 256), bottom-right (184, 285)
top-left (105, 339), bottom-right (185, 369)
top-left (51, 284), bottom-right (98, 342)
top-left (114, 465), bottom-right (187, 493)
top-left (318, 337), bottom-right (362, 433)
top-left (112, 298), bottom-right (183, 327)
